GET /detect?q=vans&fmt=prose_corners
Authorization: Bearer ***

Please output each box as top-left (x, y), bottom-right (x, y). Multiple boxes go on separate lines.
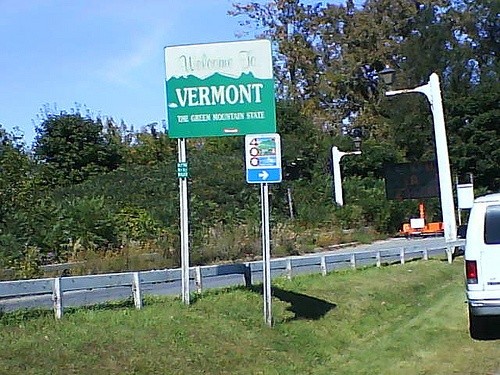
top-left (458, 193), bottom-right (500, 339)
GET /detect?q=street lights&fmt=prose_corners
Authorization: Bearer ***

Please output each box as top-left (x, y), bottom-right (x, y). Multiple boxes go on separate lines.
top-left (377, 67), bottom-right (461, 258)
top-left (331, 136), bottom-right (363, 207)
